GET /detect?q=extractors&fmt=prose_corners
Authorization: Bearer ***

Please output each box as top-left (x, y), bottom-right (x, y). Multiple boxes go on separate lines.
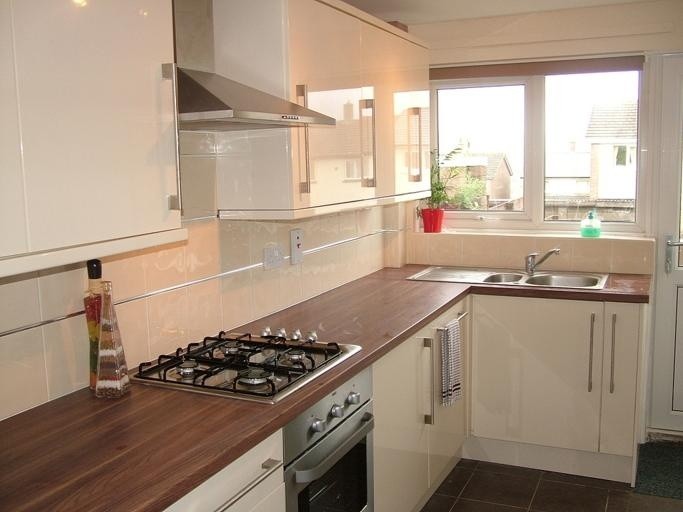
top-left (178, 66), bottom-right (336, 134)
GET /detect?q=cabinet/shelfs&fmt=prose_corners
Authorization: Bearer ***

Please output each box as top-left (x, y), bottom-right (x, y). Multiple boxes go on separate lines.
top-left (287, 2), bottom-right (427, 209)
top-left (371, 299), bottom-right (465, 512)
top-left (0, 0), bottom-right (183, 260)
top-left (470, 296), bottom-right (641, 458)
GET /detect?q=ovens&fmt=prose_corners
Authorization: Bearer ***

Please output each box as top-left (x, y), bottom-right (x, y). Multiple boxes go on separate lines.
top-left (283, 397), bottom-right (375, 510)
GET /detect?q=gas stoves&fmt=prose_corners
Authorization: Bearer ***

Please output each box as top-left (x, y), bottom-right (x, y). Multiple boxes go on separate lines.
top-left (134, 325), bottom-right (361, 407)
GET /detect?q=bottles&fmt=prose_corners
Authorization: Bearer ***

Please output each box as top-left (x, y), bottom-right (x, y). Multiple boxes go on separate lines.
top-left (579, 213), bottom-right (601, 236)
top-left (82, 260), bottom-right (102, 390)
top-left (94, 281), bottom-right (131, 398)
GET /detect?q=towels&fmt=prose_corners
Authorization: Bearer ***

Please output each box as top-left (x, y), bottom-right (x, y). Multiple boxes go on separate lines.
top-left (440, 320), bottom-right (462, 405)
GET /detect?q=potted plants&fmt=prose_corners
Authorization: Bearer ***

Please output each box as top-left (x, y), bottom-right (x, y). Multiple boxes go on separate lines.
top-left (414, 146), bottom-right (483, 233)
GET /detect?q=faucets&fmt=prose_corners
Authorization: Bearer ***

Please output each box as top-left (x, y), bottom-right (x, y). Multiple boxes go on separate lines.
top-left (525, 249), bottom-right (561, 274)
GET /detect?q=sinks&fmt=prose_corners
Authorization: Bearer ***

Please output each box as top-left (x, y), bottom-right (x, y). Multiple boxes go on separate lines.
top-left (482, 273), bottom-right (522, 283)
top-left (525, 272), bottom-right (610, 291)
